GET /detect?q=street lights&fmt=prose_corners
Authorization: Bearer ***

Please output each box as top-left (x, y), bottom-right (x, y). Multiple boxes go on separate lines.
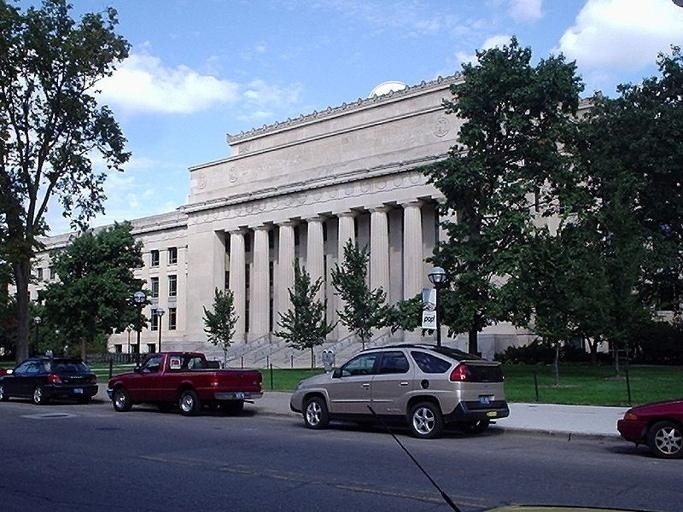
top-left (156, 308), bottom-right (166, 353)
top-left (32, 316), bottom-right (43, 355)
top-left (133, 290), bottom-right (147, 372)
top-left (54, 328), bottom-right (60, 354)
top-left (126, 328), bottom-right (133, 355)
top-left (427, 267), bottom-right (449, 346)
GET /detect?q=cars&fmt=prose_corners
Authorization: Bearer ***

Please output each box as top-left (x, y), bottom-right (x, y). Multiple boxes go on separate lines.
top-left (0, 367), bottom-right (5, 377)
top-left (0, 356), bottom-right (102, 407)
top-left (616, 398), bottom-right (682, 460)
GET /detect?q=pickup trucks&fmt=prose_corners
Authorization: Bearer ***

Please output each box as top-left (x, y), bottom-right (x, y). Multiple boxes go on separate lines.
top-left (106, 353), bottom-right (265, 419)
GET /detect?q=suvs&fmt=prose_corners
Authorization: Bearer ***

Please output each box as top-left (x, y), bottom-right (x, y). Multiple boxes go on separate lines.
top-left (289, 341), bottom-right (511, 439)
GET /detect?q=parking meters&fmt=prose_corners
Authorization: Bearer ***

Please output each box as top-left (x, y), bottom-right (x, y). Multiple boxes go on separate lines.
top-left (320, 348), bottom-right (334, 374)
